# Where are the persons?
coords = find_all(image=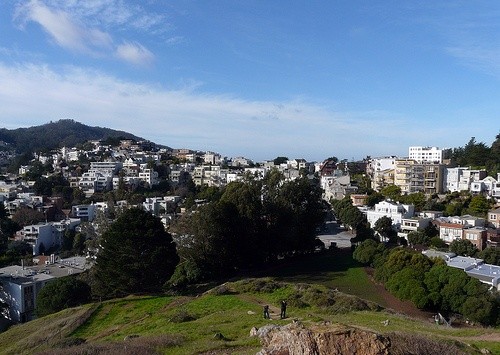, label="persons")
[280,301,287,319]
[263,305,270,319]
